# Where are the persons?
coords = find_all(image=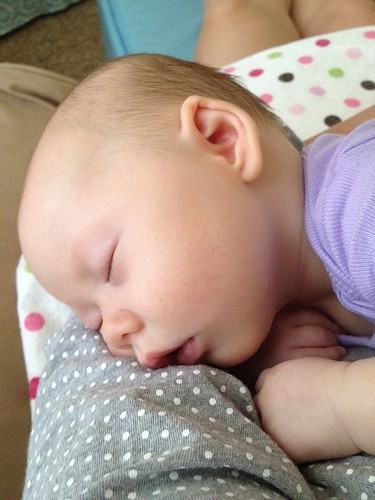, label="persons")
[16,52,375,463]
[19,2,375,500]
